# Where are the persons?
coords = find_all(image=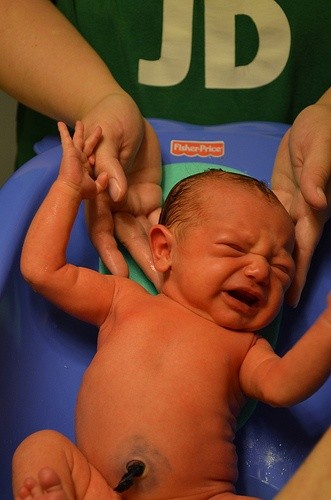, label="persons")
[12,119,331,500]
[0,0,331,307]
